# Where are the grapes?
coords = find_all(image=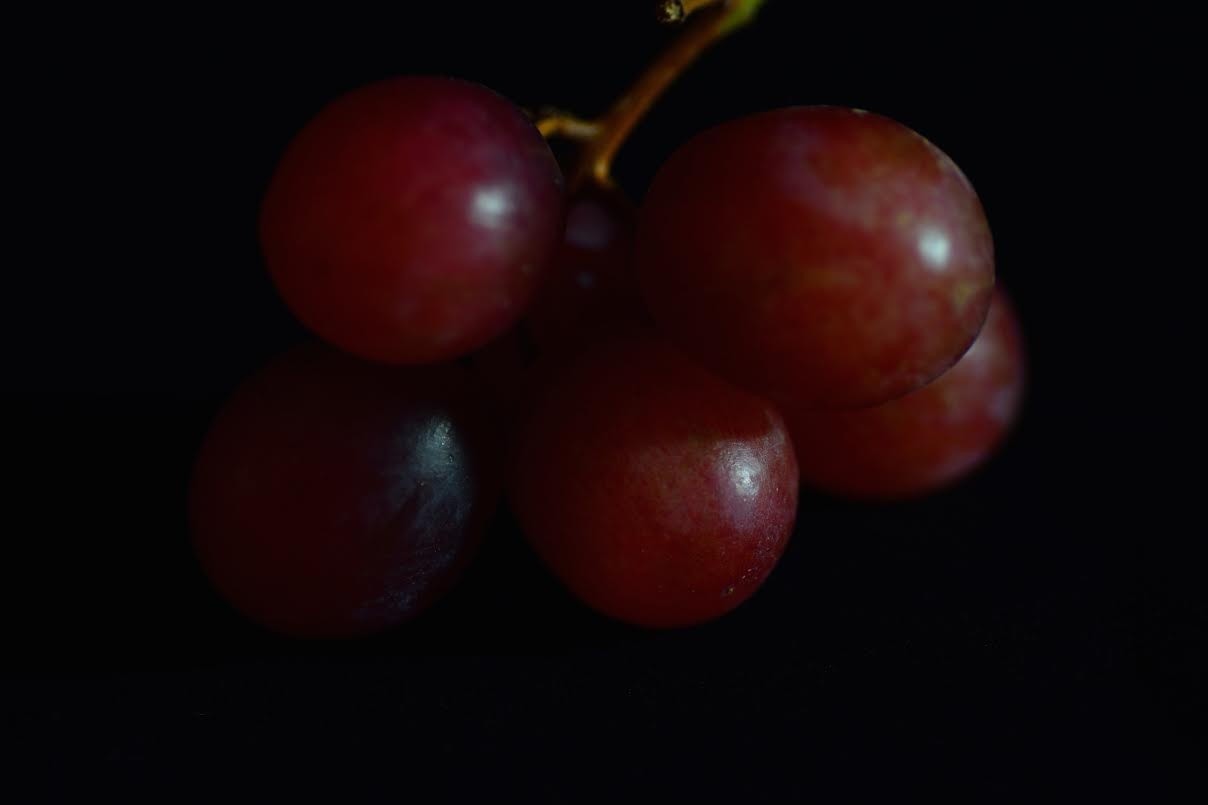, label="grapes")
[187,77,1033,642]
